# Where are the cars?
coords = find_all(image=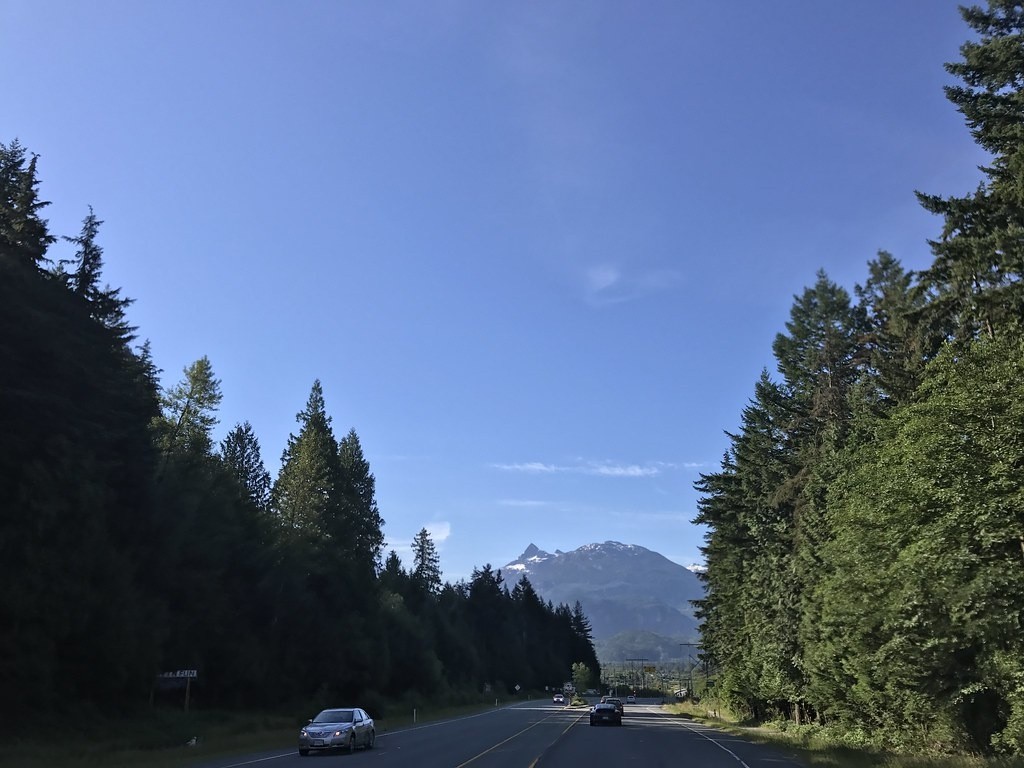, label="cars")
[600,696,612,704]
[553,694,565,703]
[589,703,622,727]
[605,698,624,716]
[297,707,376,756]
[627,696,635,704]
